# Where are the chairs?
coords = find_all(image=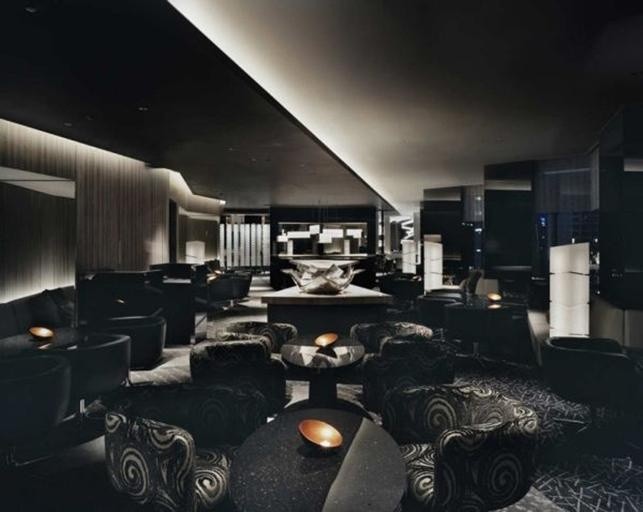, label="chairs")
[1,262,253,481]
[94,317,545,511]
[354,261,634,510]
